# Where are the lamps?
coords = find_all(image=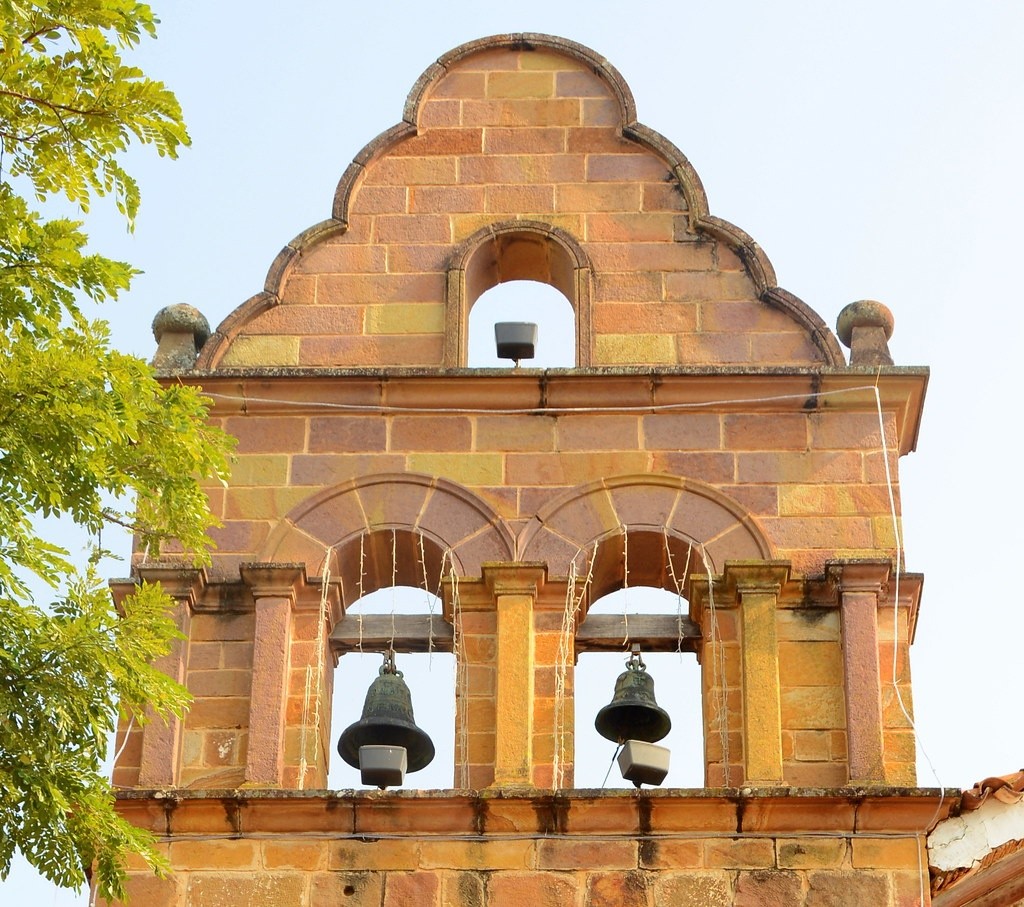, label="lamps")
[616,740,672,787]
[357,743,409,788]
[494,322,538,368]
